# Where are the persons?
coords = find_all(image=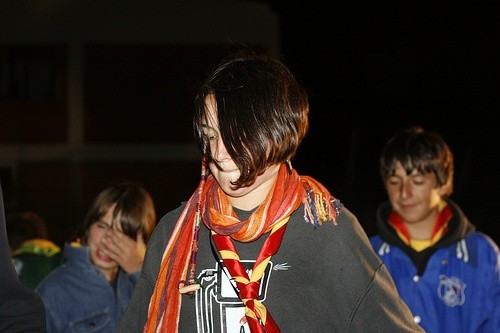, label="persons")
[369,132,500,333]
[34,182,156,333]
[6,212,65,292]
[117,57,427,333]
